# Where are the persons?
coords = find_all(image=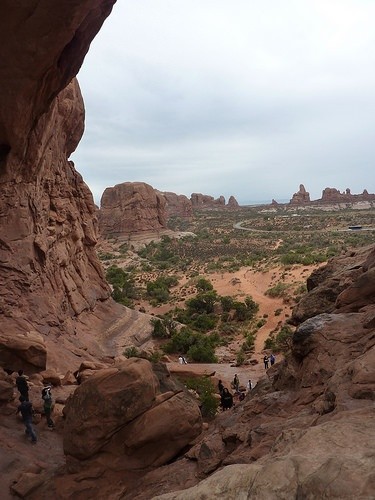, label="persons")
[16,370,55,443]
[178,355,275,411]
